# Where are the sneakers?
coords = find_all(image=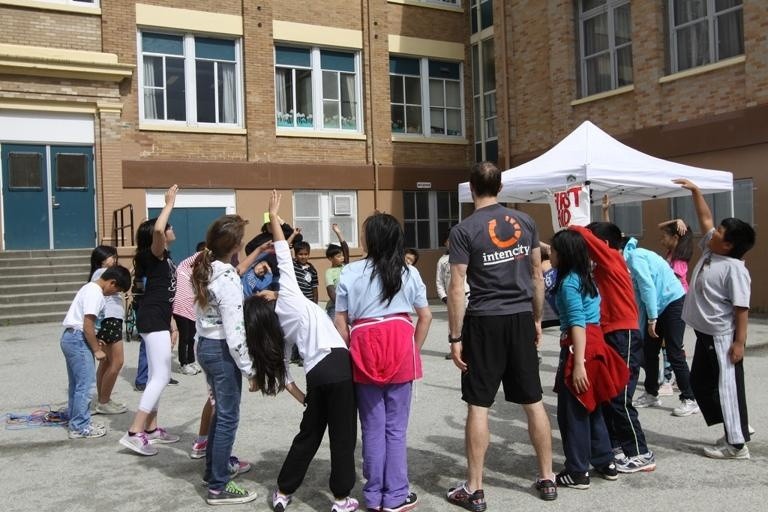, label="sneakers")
[703,423,756,459]
[445,482,488,511]
[270,488,293,512]
[533,471,559,501]
[329,496,360,512]
[135,380,148,391]
[671,397,700,417]
[205,481,259,506]
[629,392,662,409]
[176,360,203,376]
[368,492,418,511]
[610,444,656,474]
[202,455,252,483]
[553,468,590,491]
[166,379,180,386]
[95,392,128,415]
[67,414,106,439]
[189,439,208,461]
[659,380,679,395]
[590,460,618,482]
[119,425,181,457]
